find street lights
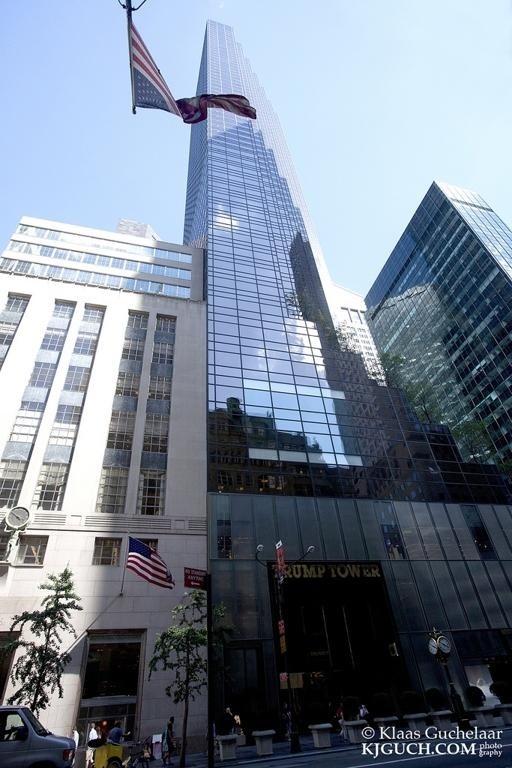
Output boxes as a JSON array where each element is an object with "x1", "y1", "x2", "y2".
[{"x1": 255, "y1": 539, "x2": 318, "y2": 758}]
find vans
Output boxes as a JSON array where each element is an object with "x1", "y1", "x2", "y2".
[{"x1": 0, "y1": 703, "x2": 76, "y2": 767}]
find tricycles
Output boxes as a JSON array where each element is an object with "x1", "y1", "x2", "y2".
[{"x1": 90, "y1": 731, "x2": 152, "y2": 767}]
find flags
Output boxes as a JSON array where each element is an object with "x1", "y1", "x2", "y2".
[{"x1": 125, "y1": 535, "x2": 175, "y2": 592}]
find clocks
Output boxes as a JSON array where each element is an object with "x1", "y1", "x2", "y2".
[
  {"x1": 5, "y1": 506, "x2": 30, "y2": 528},
  {"x1": 427, "y1": 639, "x2": 438, "y2": 656},
  {"x1": 438, "y1": 635, "x2": 452, "y2": 654}
]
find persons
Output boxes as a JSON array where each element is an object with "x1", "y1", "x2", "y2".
[
  {"x1": 72, "y1": 717, "x2": 176, "y2": 768},
  {"x1": 281, "y1": 702, "x2": 294, "y2": 741},
  {"x1": 335, "y1": 702, "x2": 346, "y2": 737},
  {"x1": 359, "y1": 704, "x2": 368, "y2": 720},
  {"x1": 223, "y1": 707, "x2": 243, "y2": 735}
]
